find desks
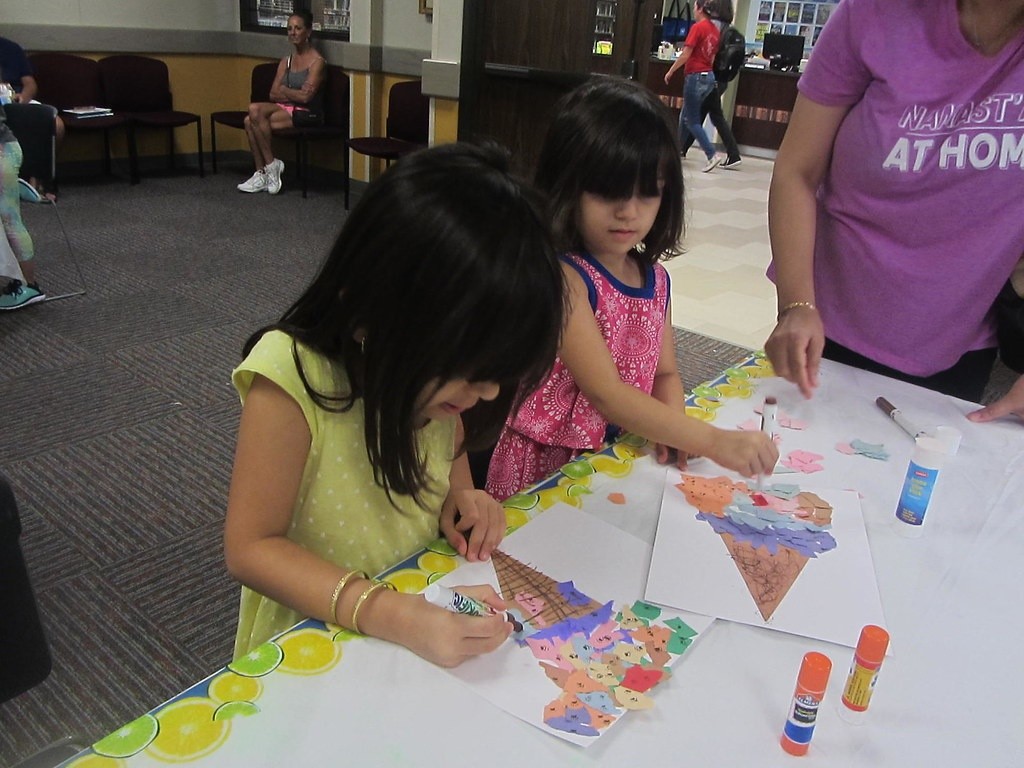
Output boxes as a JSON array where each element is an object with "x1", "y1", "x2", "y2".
[{"x1": 57, "y1": 348, "x2": 1024, "y2": 767}]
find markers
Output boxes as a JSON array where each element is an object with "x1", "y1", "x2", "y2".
[
  {"x1": 425, "y1": 584, "x2": 523, "y2": 633},
  {"x1": 754, "y1": 397, "x2": 778, "y2": 489}
]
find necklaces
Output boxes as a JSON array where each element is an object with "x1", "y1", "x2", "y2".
[{"x1": 971, "y1": 0, "x2": 980, "y2": 47}]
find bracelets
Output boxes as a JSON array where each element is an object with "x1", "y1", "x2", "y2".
[
  {"x1": 352, "y1": 581, "x2": 397, "y2": 637},
  {"x1": 776, "y1": 302, "x2": 816, "y2": 322},
  {"x1": 330, "y1": 568, "x2": 370, "y2": 626}
]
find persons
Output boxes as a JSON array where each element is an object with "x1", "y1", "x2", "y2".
[
  {"x1": 483, "y1": 76, "x2": 779, "y2": 503},
  {"x1": 665, "y1": 0, "x2": 721, "y2": 172},
  {"x1": 224, "y1": 138, "x2": 573, "y2": 667},
  {"x1": 0, "y1": 99, "x2": 46, "y2": 309},
  {"x1": 765, "y1": 0, "x2": 1024, "y2": 425},
  {"x1": 0, "y1": 37, "x2": 64, "y2": 202},
  {"x1": 237, "y1": 10, "x2": 325, "y2": 195},
  {"x1": 680, "y1": 0, "x2": 741, "y2": 168}
]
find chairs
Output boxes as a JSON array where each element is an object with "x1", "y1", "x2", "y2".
[
  {"x1": 2, "y1": 54, "x2": 202, "y2": 305},
  {"x1": 210, "y1": 63, "x2": 430, "y2": 210}
]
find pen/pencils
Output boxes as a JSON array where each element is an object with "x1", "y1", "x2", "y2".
[{"x1": 876, "y1": 395, "x2": 926, "y2": 440}]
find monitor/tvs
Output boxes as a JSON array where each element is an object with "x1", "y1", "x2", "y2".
[{"x1": 763, "y1": 33, "x2": 805, "y2": 70}]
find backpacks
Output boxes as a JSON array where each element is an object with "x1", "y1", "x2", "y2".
[{"x1": 712, "y1": 26, "x2": 745, "y2": 83}]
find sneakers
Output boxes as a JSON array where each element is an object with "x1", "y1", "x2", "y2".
[
  {"x1": 237, "y1": 169, "x2": 269, "y2": 193},
  {"x1": 265, "y1": 158, "x2": 285, "y2": 195},
  {"x1": 17, "y1": 178, "x2": 41, "y2": 203},
  {"x1": 718, "y1": 154, "x2": 743, "y2": 168},
  {"x1": 679, "y1": 150, "x2": 688, "y2": 159},
  {"x1": 0, "y1": 280, "x2": 47, "y2": 310},
  {"x1": 701, "y1": 154, "x2": 721, "y2": 173}
]
